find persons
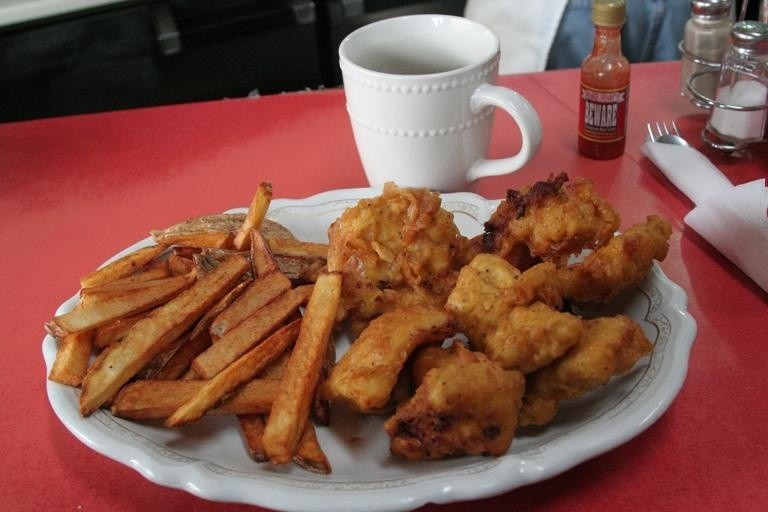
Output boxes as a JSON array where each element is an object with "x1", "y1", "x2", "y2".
[{"x1": 462, "y1": 0, "x2": 692, "y2": 74}]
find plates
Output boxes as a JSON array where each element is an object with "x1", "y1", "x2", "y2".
[{"x1": 43, "y1": 186, "x2": 699, "y2": 512}]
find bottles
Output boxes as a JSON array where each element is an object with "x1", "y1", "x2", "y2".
[
  {"x1": 577, "y1": 1, "x2": 630, "y2": 161},
  {"x1": 682, "y1": 0, "x2": 768, "y2": 144}
]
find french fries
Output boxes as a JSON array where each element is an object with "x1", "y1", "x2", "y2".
[{"x1": 43, "y1": 181, "x2": 344, "y2": 475}]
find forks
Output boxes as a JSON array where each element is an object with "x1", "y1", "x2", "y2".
[{"x1": 646, "y1": 119, "x2": 680, "y2": 143}]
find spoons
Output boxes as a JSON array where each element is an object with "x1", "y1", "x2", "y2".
[{"x1": 659, "y1": 136, "x2": 690, "y2": 149}]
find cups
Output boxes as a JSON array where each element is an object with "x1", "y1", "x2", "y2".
[{"x1": 337, "y1": 14, "x2": 542, "y2": 196}]
